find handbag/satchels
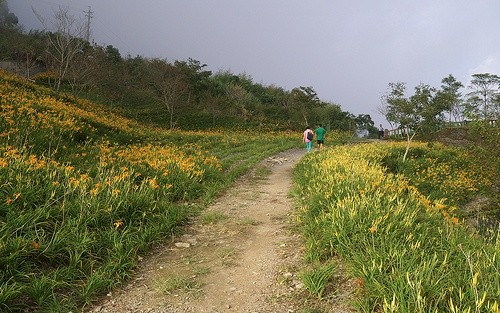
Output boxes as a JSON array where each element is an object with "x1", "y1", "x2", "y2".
[{"x1": 307, "y1": 132, "x2": 314, "y2": 141}]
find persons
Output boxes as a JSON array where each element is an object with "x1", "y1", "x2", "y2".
[
  {"x1": 302, "y1": 126, "x2": 314, "y2": 152},
  {"x1": 377, "y1": 124, "x2": 385, "y2": 139},
  {"x1": 314, "y1": 123, "x2": 326, "y2": 150}
]
